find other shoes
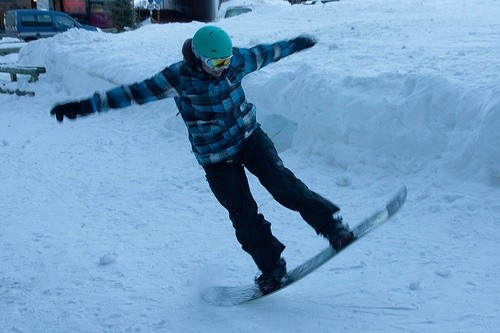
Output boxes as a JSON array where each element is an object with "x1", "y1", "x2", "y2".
[
  {"x1": 320, "y1": 214, "x2": 354, "y2": 253},
  {"x1": 254, "y1": 258, "x2": 288, "y2": 296}
]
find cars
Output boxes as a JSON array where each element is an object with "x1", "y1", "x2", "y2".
[{"x1": 224, "y1": 6, "x2": 253, "y2": 19}]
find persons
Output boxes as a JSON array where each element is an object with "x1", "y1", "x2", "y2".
[{"x1": 51, "y1": 25, "x2": 354, "y2": 295}]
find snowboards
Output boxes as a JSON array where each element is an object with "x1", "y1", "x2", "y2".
[{"x1": 197, "y1": 184, "x2": 409, "y2": 307}]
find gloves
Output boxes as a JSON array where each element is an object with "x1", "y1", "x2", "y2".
[
  {"x1": 50, "y1": 98, "x2": 94, "y2": 121},
  {"x1": 289, "y1": 36, "x2": 316, "y2": 53}
]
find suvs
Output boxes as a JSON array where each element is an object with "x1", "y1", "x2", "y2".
[{"x1": 4, "y1": 9, "x2": 103, "y2": 42}]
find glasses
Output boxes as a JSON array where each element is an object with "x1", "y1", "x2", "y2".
[{"x1": 201, "y1": 55, "x2": 233, "y2": 71}]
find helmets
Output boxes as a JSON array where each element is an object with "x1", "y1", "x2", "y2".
[{"x1": 192, "y1": 25, "x2": 233, "y2": 60}]
